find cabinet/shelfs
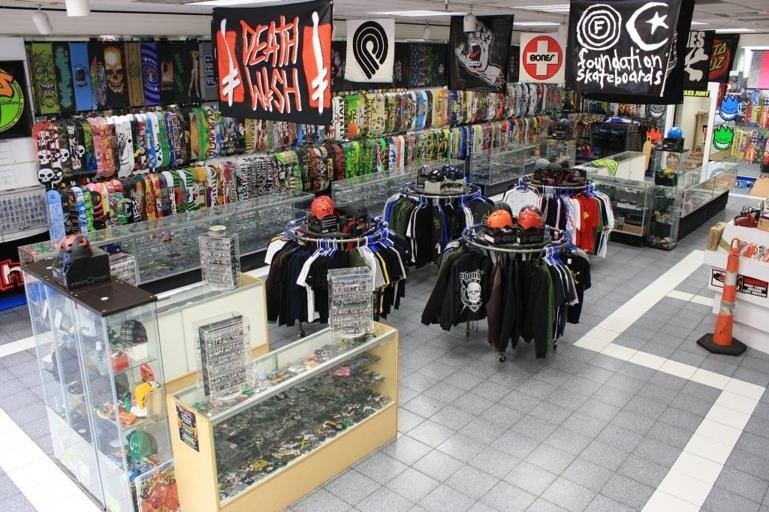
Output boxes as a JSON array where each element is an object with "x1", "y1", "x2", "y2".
[
  {"x1": 702, "y1": 219, "x2": 769, "y2": 356},
  {"x1": 18, "y1": 228, "x2": 139, "y2": 287},
  {"x1": 645, "y1": 187, "x2": 682, "y2": 251},
  {"x1": 573, "y1": 151, "x2": 645, "y2": 181},
  {"x1": 677, "y1": 161, "x2": 739, "y2": 242},
  {"x1": 166, "y1": 321, "x2": 399, "y2": 511},
  {"x1": 654, "y1": 147, "x2": 689, "y2": 187},
  {"x1": 588, "y1": 175, "x2": 654, "y2": 249},
  {"x1": 470, "y1": 142, "x2": 541, "y2": 196},
  {"x1": 330, "y1": 160, "x2": 466, "y2": 216},
  {"x1": 156, "y1": 272, "x2": 270, "y2": 393},
  {"x1": 21, "y1": 261, "x2": 179, "y2": 512},
  {"x1": 116, "y1": 191, "x2": 315, "y2": 285}
]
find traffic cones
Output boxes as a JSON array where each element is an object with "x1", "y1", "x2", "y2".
[{"x1": 692, "y1": 237, "x2": 748, "y2": 356}]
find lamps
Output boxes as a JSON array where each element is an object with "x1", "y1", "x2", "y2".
[
  {"x1": 66, "y1": 0, "x2": 91, "y2": 16},
  {"x1": 463, "y1": 12, "x2": 477, "y2": 34},
  {"x1": 34, "y1": 13, "x2": 52, "y2": 34}
]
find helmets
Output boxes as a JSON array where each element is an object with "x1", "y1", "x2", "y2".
[
  {"x1": 517, "y1": 211, "x2": 544, "y2": 229},
  {"x1": 344, "y1": 202, "x2": 369, "y2": 220},
  {"x1": 557, "y1": 118, "x2": 570, "y2": 129},
  {"x1": 520, "y1": 204, "x2": 542, "y2": 217},
  {"x1": 760, "y1": 154, "x2": 769, "y2": 172},
  {"x1": 428, "y1": 168, "x2": 445, "y2": 182},
  {"x1": 486, "y1": 202, "x2": 513, "y2": 217},
  {"x1": 535, "y1": 158, "x2": 551, "y2": 171},
  {"x1": 547, "y1": 162, "x2": 563, "y2": 173},
  {"x1": 57, "y1": 233, "x2": 92, "y2": 264},
  {"x1": 668, "y1": 126, "x2": 682, "y2": 139},
  {"x1": 446, "y1": 167, "x2": 464, "y2": 179},
  {"x1": 310, "y1": 195, "x2": 335, "y2": 220},
  {"x1": 441, "y1": 164, "x2": 454, "y2": 175},
  {"x1": 486, "y1": 209, "x2": 513, "y2": 228},
  {"x1": 419, "y1": 164, "x2": 433, "y2": 178}
]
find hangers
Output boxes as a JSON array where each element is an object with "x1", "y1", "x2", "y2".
[
  {"x1": 400, "y1": 182, "x2": 489, "y2": 211},
  {"x1": 285, "y1": 216, "x2": 397, "y2": 256},
  {"x1": 457, "y1": 222, "x2": 572, "y2": 265},
  {"x1": 518, "y1": 172, "x2": 596, "y2": 199}
]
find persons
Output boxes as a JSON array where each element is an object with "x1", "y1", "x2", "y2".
[{"x1": 188, "y1": 51, "x2": 200, "y2": 98}]
